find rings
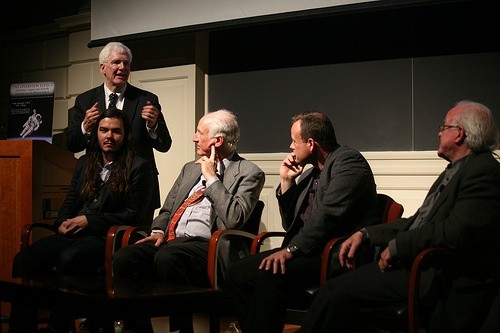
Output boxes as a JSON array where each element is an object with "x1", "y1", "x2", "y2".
[{"x1": 274, "y1": 257, "x2": 279, "y2": 262}]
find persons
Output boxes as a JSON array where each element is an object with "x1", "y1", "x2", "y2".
[
  {"x1": 111, "y1": 109, "x2": 265, "y2": 333},
  {"x1": 225, "y1": 111, "x2": 378, "y2": 333},
  {"x1": 8, "y1": 110, "x2": 156, "y2": 333},
  {"x1": 295, "y1": 100, "x2": 500, "y2": 333},
  {"x1": 64, "y1": 41, "x2": 172, "y2": 230}
]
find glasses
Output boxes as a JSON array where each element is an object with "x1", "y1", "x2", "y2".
[{"x1": 438, "y1": 124, "x2": 458, "y2": 132}]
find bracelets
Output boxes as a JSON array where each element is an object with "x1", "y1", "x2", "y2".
[{"x1": 286, "y1": 244, "x2": 299, "y2": 253}]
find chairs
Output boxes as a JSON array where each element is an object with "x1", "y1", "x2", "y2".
[{"x1": 20, "y1": 193, "x2": 460, "y2": 333}]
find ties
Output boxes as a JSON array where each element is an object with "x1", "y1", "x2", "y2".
[
  {"x1": 107, "y1": 93, "x2": 119, "y2": 109},
  {"x1": 164, "y1": 188, "x2": 207, "y2": 242}
]
